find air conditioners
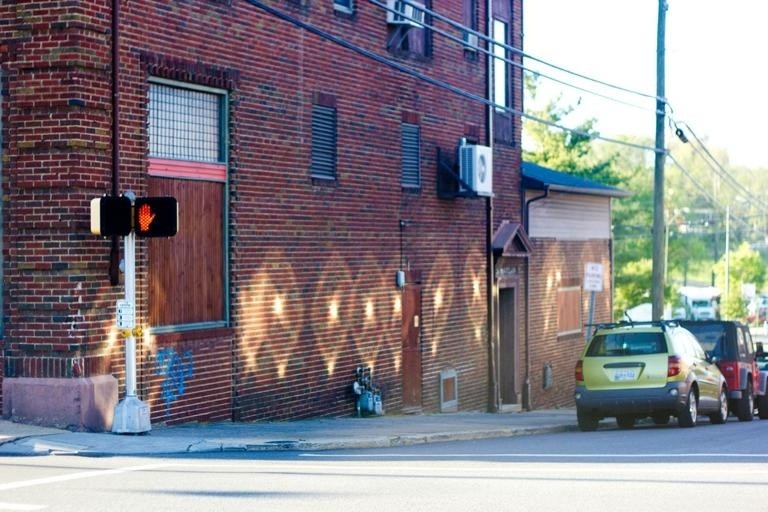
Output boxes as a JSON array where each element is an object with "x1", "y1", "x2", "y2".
[
  {"x1": 459, "y1": 143, "x2": 494, "y2": 193},
  {"x1": 386, "y1": 0, "x2": 426, "y2": 29}
]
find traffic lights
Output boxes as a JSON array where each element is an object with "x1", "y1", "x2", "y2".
[{"x1": 89, "y1": 196, "x2": 179, "y2": 237}]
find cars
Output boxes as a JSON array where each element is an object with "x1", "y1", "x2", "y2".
[{"x1": 575, "y1": 319, "x2": 768, "y2": 427}]
